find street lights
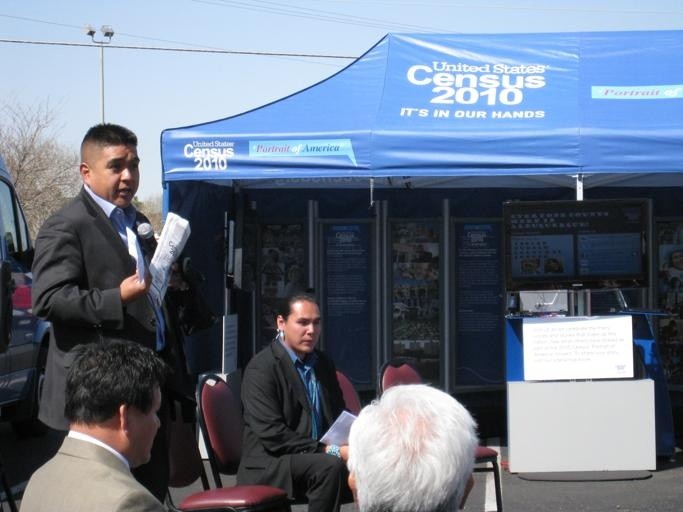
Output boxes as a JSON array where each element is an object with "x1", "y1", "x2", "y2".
[{"x1": 86, "y1": 23, "x2": 113, "y2": 125}]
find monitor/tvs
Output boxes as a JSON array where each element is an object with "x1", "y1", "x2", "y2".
[
  {"x1": 228, "y1": 190, "x2": 259, "y2": 292},
  {"x1": 501, "y1": 197, "x2": 654, "y2": 291}
]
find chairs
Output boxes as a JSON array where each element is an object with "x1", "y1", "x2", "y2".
[{"x1": 158, "y1": 362, "x2": 501, "y2": 512}]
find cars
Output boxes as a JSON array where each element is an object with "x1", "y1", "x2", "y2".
[{"x1": 0, "y1": 156, "x2": 51, "y2": 435}]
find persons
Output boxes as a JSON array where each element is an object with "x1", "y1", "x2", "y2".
[
  {"x1": 32, "y1": 124, "x2": 182, "y2": 505},
  {"x1": 18, "y1": 342, "x2": 170, "y2": 511},
  {"x1": 657, "y1": 244, "x2": 683, "y2": 386},
  {"x1": 346, "y1": 383, "x2": 481, "y2": 511},
  {"x1": 236, "y1": 292, "x2": 349, "y2": 512}
]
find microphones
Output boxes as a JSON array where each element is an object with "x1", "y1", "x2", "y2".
[{"x1": 137, "y1": 222, "x2": 157, "y2": 253}]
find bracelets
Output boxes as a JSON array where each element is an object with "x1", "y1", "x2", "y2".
[{"x1": 326, "y1": 444, "x2": 341, "y2": 457}]
